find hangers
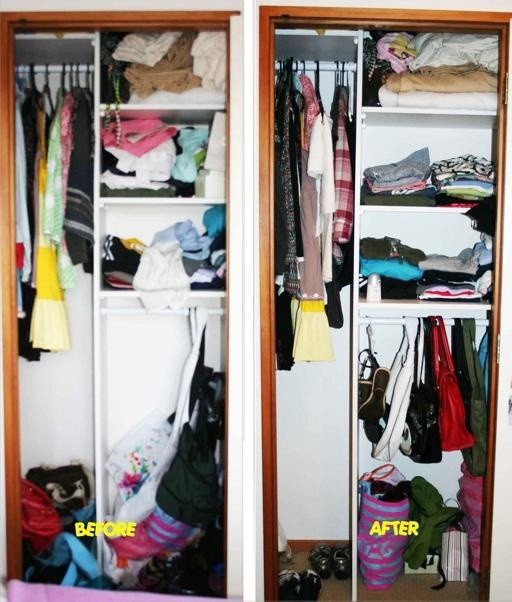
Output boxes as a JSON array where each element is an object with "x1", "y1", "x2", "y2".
[
  {"x1": 13, "y1": 62, "x2": 94, "y2": 129},
  {"x1": 273, "y1": 57, "x2": 356, "y2": 138}
]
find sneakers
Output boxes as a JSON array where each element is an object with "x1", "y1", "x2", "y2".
[
  {"x1": 309, "y1": 544, "x2": 332, "y2": 577},
  {"x1": 335, "y1": 543, "x2": 351, "y2": 579},
  {"x1": 278, "y1": 569, "x2": 299, "y2": 600},
  {"x1": 301, "y1": 570, "x2": 321, "y2": 600}
]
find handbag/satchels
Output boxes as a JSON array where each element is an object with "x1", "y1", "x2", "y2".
[
  {"x1": 442, "y1": 521, "x2": 469, "y2": 583},
  {"x1": 145, "y1": 505, "x2": 193, "y2": 547},
  {"x1": 402, "y1": 478, "x2": 465, "y2": 590},
  {"x1": 462, "y1": 317, "x2": 487, "y2": 476},
  {"x1": 354, "y1": 347, "x2": 390, "y2": 422},
  {"x1": 155, "y1": 321, "x2": 221, "y2": 521},
  {"x1": 454, "y1": 462, "x2": 482, "y2": 575},
  {"x1": 105, "y1": 410, "x2": 177, "y2": 500},
  {"x1": 430, "y1": 316, "x2": 475, "y2": 452},
  {"x1": 357, "y1": 494, "x2": 409, "y2": 590},
  {"x1": 410, "y1": 317, "x2": 442, "y2": 464},
  {"x1": 374, "y1": 316, "x2": 419, "y2": 463},
  {"x1": 23, "y1": 460, "x2": 90, "y2": 512}
]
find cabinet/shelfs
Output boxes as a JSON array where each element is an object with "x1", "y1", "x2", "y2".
[
  {"x1": 265, "y1": 8, "x2": 509, "y2": 601},
  {"x1": 8, "y1": 12, "x2": 240, "y2": 601}
]
